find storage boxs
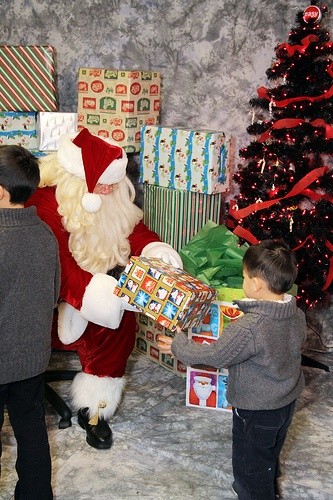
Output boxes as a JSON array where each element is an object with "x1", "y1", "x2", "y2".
[
  {"x1": 142, "y1": 184, "x2": 222, "y2": 254},
  {"x1": 0, "y1": 44, "x2": 60, "y2": 112},
  {"x1": 77, "y1": 67, "x2": 162, "y2": 153},
  {"x1": 137, "y1": 125, "x2": 231, "y2": 196},
  {"x1": 1, "y1": 108, "x2": 49, "y2": 158},
  {"x1": 112, "y1": 256, "x2": 220, "y2": 334},
  {"x1": 37, "y1": 111, "x2": 78, "y2": 151}
]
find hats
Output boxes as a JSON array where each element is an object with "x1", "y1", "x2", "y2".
[{"x1": 57, "y1": 128, "x2": 128, "y2": 213}]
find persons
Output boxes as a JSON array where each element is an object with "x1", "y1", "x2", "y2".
[
  {"x1": 156, "y1": 238, "x2": 307, "y2": 500},
  {"x1": 23, "y1": 128, "x2": 180, "y2": 452},
  {"x1": 0, "y1": 145, "x2": 59, "y2": 499}
]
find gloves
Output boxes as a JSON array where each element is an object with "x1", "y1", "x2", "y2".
[
  {"x1": 122, "y1": 299, "x2": 139, "y2": 314},
  {"x1": 142, "y1": 242, "x2": 183, "y2": 270}
]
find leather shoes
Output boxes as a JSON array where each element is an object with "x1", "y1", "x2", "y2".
[{"x1": 77, "y1": 404, "x2": 113, "y2": 448}]
